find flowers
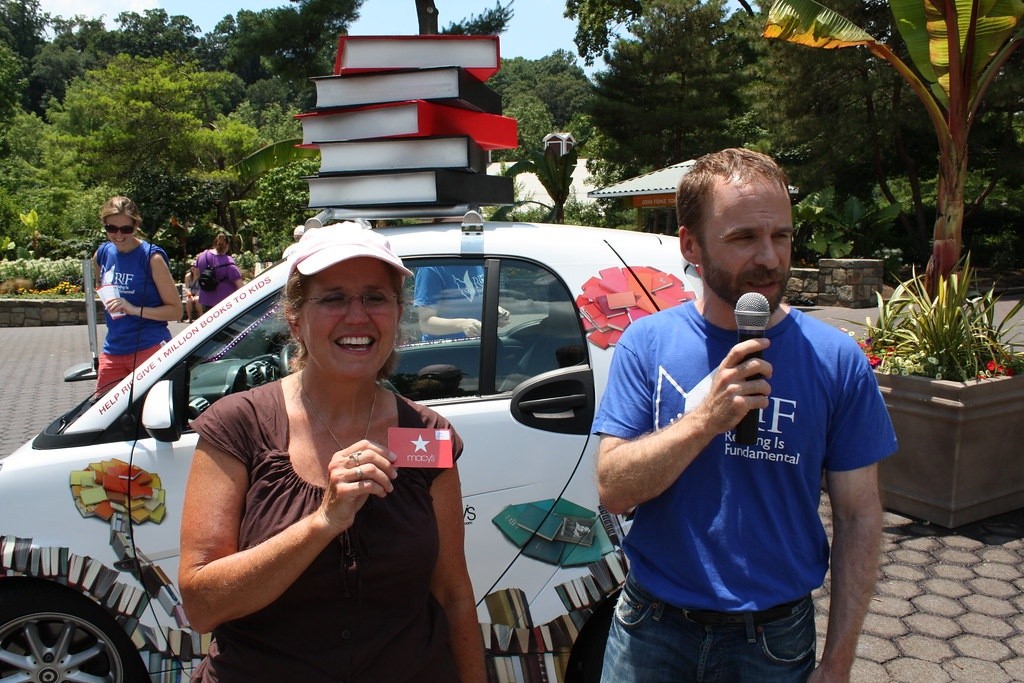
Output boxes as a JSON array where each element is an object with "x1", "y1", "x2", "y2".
[{"x1": 761, "y1": 1, "x2": 1023, "y2": 387}]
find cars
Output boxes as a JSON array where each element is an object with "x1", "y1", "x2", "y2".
[{"x1": 1, "y1": 208, "x2": 710, "y2": 683}]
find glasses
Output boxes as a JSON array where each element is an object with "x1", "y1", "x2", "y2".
[{"x1": 104, "y1": 223, "x2": 137, "y2": 234}]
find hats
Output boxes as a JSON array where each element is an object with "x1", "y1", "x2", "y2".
[
  {"x1": 285, "y1": 219, "x2": 415, "y2": 296},
  {"x1": 293, "y1": 224, "x2": 304, "y2": 235}
]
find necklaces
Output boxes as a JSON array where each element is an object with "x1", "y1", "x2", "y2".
[{"x1": 301, "y1": 371, "x2": 377, "y2": 449}]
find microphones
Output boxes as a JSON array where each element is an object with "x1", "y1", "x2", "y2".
[{"x1": 734, "y1": 292, "x2": 771, "y2": 446}]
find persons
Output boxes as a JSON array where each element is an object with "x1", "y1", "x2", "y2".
[
  {"x1": 413, "y1": 218, "x2": 510, "y2": 340},
  {"x1": 184, "y1": 261, "x2": 203, "y2": 323},
  {"x1": 94, "y1": 196, "x2": 183, "y2": 397},
  {"x1": 283, "y1": 225, "x2": 305, "y2": 258},
  {"x1": 179, "y1": 222, "x2": 488, "y2": 683},
  {"x1": 591, "y1": 149, "x2": 900, "y2": 683},
  {"x1": 419, "y1": 364, "x2": 467, "y2": 396},
  {"x1": 195, "y1": 233, "x2": 243, "y2": 313}
]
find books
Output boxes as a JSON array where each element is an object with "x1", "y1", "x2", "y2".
[{"x1": 294, "y1": 34, "x2": 518, "y2": 209}]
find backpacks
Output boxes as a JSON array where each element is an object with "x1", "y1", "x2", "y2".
[{"x1": 198, "y1": 250, "x2": 236, "y2": 291}]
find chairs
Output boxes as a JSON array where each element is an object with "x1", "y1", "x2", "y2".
[{"x1": 499, "y1": 305, "x2": 586, "y2": 394}]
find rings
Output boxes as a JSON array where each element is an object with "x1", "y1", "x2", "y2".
[
  {"x1": 349, "y1": 451, "x2": 361, "y2": 466},
  {"x1": 356, "y1": 466, "x2": 362, "y2": 479}
]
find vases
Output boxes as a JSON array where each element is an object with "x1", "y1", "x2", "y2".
[{"x1": 867, "y1": 369, "x2": 1024, "y2": 530}]
